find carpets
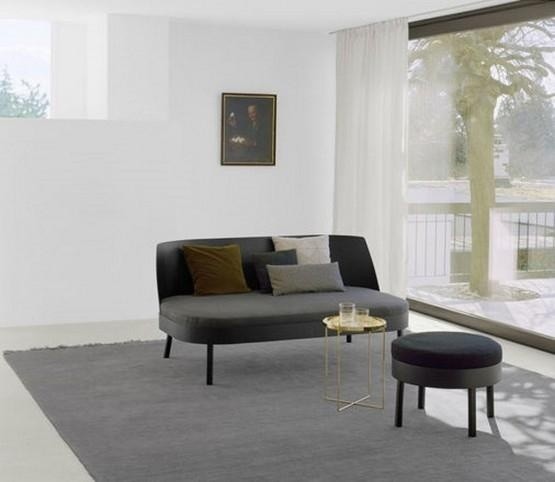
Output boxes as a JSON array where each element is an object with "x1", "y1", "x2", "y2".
[{"x1": 2, "y1": 327, "x2": 555, "y2": 482}]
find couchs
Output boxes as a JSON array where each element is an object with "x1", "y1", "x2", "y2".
[{"x1": 156, "y1": 234, "x2": 410, "y2": 386}]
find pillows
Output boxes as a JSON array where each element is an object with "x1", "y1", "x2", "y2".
[{"x1": 182, "y1": 235, "x2": 347, "y2": 297}]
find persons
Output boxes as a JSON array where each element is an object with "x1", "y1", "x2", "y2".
[{"x1": 227, "y1": 104, "x2": 267, "y2": 163}]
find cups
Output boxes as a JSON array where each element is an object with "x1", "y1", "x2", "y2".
[{"x1": 339, "y1": 303, "x2": 370, "y2": 325}]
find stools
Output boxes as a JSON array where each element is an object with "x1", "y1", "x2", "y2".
[
  {"x1": 322, "y1": 315, "x2": 388, "y2": 413},
  {"x1": 391, "y1": 331, "x2": 502, "y2": 437}
]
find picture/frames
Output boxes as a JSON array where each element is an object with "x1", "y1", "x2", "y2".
[{"x1": 221, "y1": 93, "x2": 277, "y2": 166}]
